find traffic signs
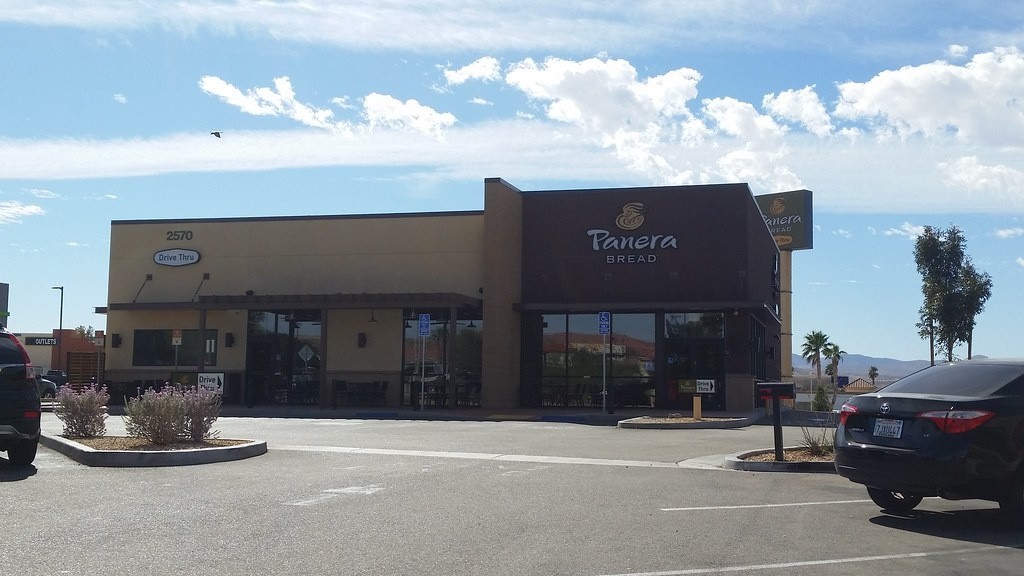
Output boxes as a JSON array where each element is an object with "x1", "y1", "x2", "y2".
[{"x1": 696, "y1": 380, "x2": 715, "y2": 393}]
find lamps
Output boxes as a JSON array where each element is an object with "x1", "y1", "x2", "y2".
[
  {"x1": 368, "y1": 302, "x2": 378, "y2": 323},
  {"x1": 112, "y1": 333, "x2": 121, "y2": 348},
  {"x1": 404, "y1": 320, "x2": 412, "y2": 329},
  {"x1": 132, "y1": 273, "x2": 153, "y2": 306},
  {"x1": 224, "y1": 333, "x2": 235, "y2": 347},
  {"x1": 190, "y1": 273, "x2": 210, "y2": 307},
  {"x1": 358, "y1": 333, "x2": 365, "y2": 346},
  {"x1": 467, "y1": 319, "x2": 477, "y2": 328}
]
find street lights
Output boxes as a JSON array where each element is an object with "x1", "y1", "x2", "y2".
[
  {"x1": 52, "y1": 285, "x2": 64, "y2": 389},
  {"x1": 808, "y1": 371, "x2": 815, "y2": 412}
]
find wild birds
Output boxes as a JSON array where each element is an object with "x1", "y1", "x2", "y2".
[{"x1": 211, "y1": 132, "x2": 222, "y2": 138}]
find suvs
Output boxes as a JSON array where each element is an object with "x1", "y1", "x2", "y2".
[{"x1": 0, "y1": 325, "x2": 43, "y2": 467}]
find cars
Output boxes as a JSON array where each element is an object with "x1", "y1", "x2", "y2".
[
  {"x1": 36, "y1": 378, "x2": 58, "y2": 398},
  {"x1": 602, "y1": 374, "x2": 653, "y2": 408},
  {"x1": 830, "y1": 357, "x2": 1024, "y2": 513},
  {"x1": 403, "y1": 358, "x2": 480, "y2": 397}
]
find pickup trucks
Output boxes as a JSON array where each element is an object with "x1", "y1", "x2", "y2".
[{"x1": 42, "y1": 370, "x2": 67, "y2": 380}]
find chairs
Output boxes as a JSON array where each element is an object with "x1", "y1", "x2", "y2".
[
  {"x1": 292, "y1": 378, "x2": 319, "y2": 406},
  {"x1": 541, "y1": 383, "x2": 638, "y2": 406},
  {"x1": 332, "y1": 380, "x2": 388, "y2": 404},
  {"x1": 410, "y1": 379, "x2": 482, "y2": 412}
]
